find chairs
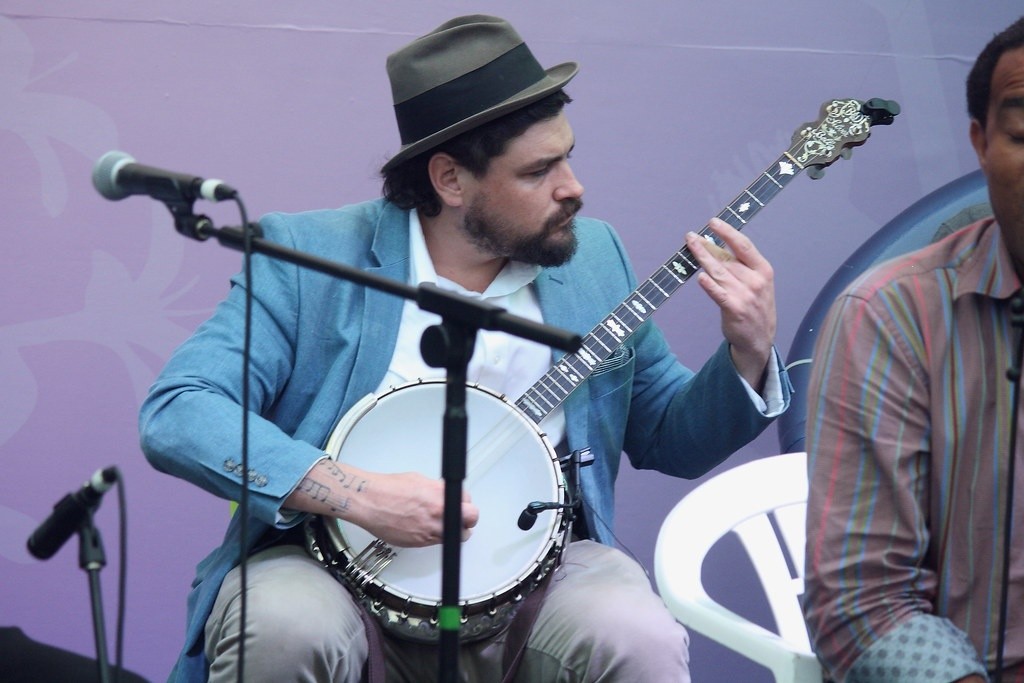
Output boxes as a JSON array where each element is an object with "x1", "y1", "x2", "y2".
[{"x1": 655, "y1": 453, "x2": 822, "y2": 683}]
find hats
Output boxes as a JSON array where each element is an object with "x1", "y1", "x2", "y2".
[{"x1": 381, "y1": 14, "x2": 579, "y2": 173}]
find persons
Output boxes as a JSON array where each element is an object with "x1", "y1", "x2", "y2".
[
  {"x1": 136, "y1": 14, "x2": 796, "y2": 682},
  {"x1": 797, "y1": 13, "x2": 1024, "y2": 683}
]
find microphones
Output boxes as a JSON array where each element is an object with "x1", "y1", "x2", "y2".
[
  {"x1": 26, "y1": 466, "x2": 118, "y2": 560},
  {"x1": 518, "y1": 501, "x2": 547, "y2": 530},
  {"x1": 92, "y1": 150, "x2": 237, "y2": 201}
]
